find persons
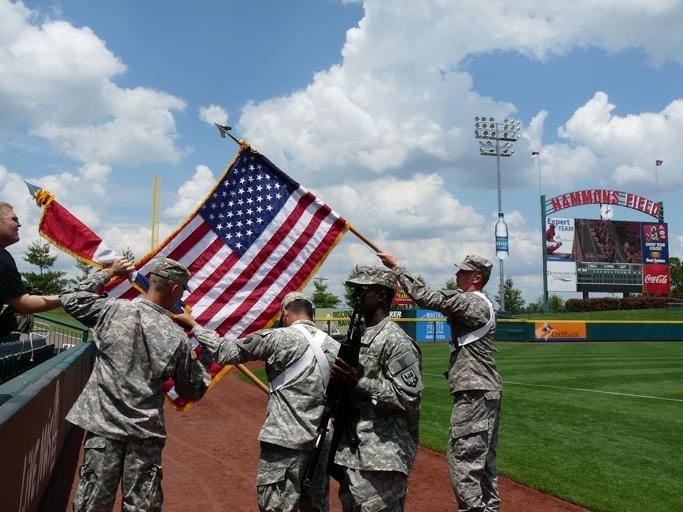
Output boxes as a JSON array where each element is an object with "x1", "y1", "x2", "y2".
[
  {"x1": 377, "y1": 250, "x2": 503, "y2": 511},
  {"x1": 330, "y1": 263, "x2": 426, "y2": 512},
  {"x1": 0, "y1": 203, "x2": 63, "y2": 344},
  {"x1": 546, "y1": 224, "x2": 562, "y2": 254},
  {"x1": 61, "y1": 256, "x2": 214, "y2": 512},
  {"x1": 172, "y1": 290, "x2": 340, "y2": 512}
]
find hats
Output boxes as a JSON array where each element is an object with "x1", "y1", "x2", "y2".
[
  {"x1": 454, "y1": 254, "x2": 493, "y2": 272},
  {"x1": 149, "y1": 257, "x2": 191, "y2": 293},
  {"x1": 279, "y1": 291, "x2": 312, "y2": 327},
  {"x1": 345, "y1": 265, "x2": 401, "y2": 291}
]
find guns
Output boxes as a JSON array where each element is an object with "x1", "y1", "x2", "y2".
[{"x1": 301, "y1": 286, "x2": 368, "y2": 488}]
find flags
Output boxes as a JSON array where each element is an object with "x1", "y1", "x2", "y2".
[
  {"x1": 91, "y1": 143, "x2": 347, "y2": 413},
  {"x1": 34, "y1": 189, "x2": 194, "y2": 338}
]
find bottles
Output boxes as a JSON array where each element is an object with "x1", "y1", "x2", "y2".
[{"x1": 494, "y1": 212, "x2": 512, "y2": 260}]
